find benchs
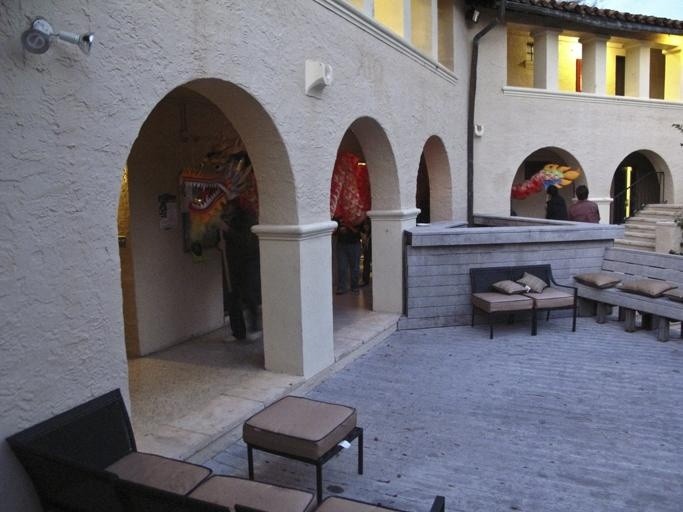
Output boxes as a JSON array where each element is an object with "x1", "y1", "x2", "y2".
[
  {"x1": 0, "y1": 388, "x2": 448, "y2": 511},
  {"x1": 573, "y1": 248, "x2": 682, "y2": 342},
  {"x1": 471, "y1": 264, "x2": 578, "y2": 339}
]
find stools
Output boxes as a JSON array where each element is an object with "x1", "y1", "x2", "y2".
[{"x1": 241, "y1": 393, "x2": 364, "y2": 501}]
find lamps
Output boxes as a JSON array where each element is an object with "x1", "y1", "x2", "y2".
[{"x1": 21, "y1": 16, "x2": 91, "y2": 56}]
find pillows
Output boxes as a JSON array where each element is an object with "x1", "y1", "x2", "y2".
[
  {"x1": 573, "y1": 272, "x2": 620, "y2": 288},
  {"x1": 616, "y1": 278, "x2": 678, "y2": 297},
  {"x1": 492, "y1": 280, "x2": 527, "y2": 294},
  {"x1": 516, "y1": 271, "x2": 547, "y2": 294},
  {"x1": 663, "y1": 287, "x2": 682, "y2": 301}
]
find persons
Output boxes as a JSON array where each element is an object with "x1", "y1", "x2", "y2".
[
  {"x1": 335, "y1": 219, "x2": 360, "y2": 294},
  {"x1": 215, "y1": 200, "x2": 263, "y2": 344},
  {"x1": 546, "y1": 185, "x2": 566, "y2": 220},
  {"x1": 358, "y1": 219, "x2": 373, "y2": 288},
  {"x1": 570, "y1": 186, "x2": 600, "y2": 224}
]
